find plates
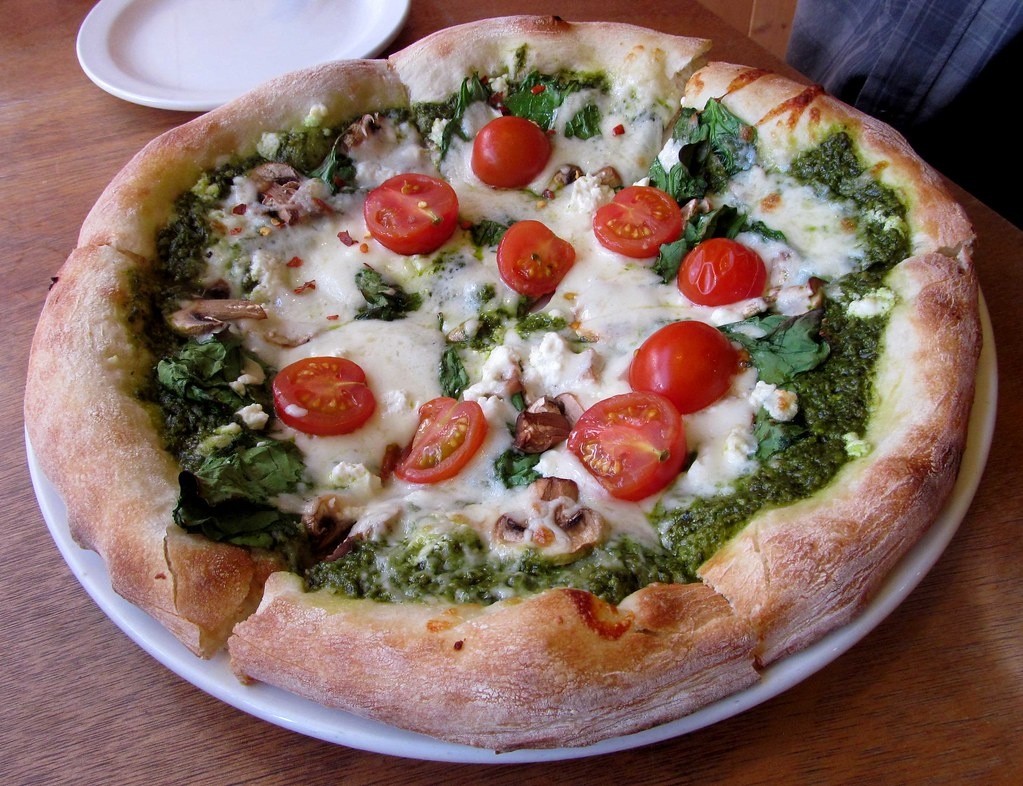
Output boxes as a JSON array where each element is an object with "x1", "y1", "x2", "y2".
[
  {"x1": 76, "y1": 0, "x2": 413, "y2": 111},
  {"x1": 24, "y1": 39, "x2": 1002, "y2": 763}
]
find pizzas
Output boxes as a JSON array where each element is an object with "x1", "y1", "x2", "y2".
[{"x1": 22, "y1": 14, "x2": 980, "y2": 752}]
